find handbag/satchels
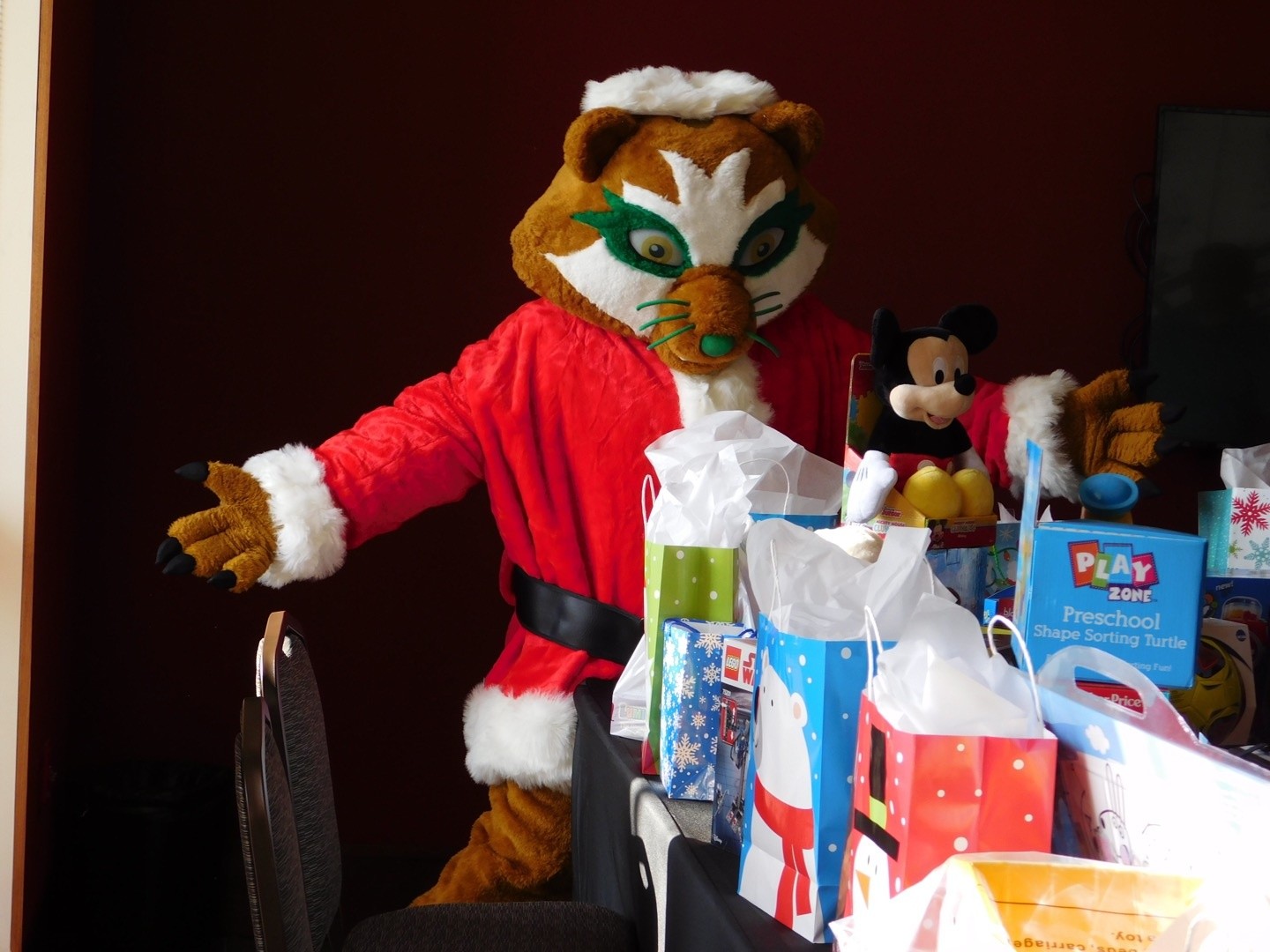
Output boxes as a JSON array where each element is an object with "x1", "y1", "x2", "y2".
[{"x1": 610, "y1": 409, "x2": 1270, "y2": 952}]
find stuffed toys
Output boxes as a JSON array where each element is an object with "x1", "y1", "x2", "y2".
[
  {"x1": 829, "y1": 297, "x2": 1011, "y2": 525},
  {"x1": 178, "y1": 48, "x2": 1168, "y2": 916}
]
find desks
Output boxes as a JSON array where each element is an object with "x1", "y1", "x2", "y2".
[{"x1": 571, "y1": 683, "x2": 833, "y2": 952}]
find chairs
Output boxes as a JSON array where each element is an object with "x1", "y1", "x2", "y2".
[
  {"x1": 255, "y1": 608, "x2": 627, "y2": 951},
  {"x1": 233, "y1": 695, "x2": 320, "y2": 951}
]
